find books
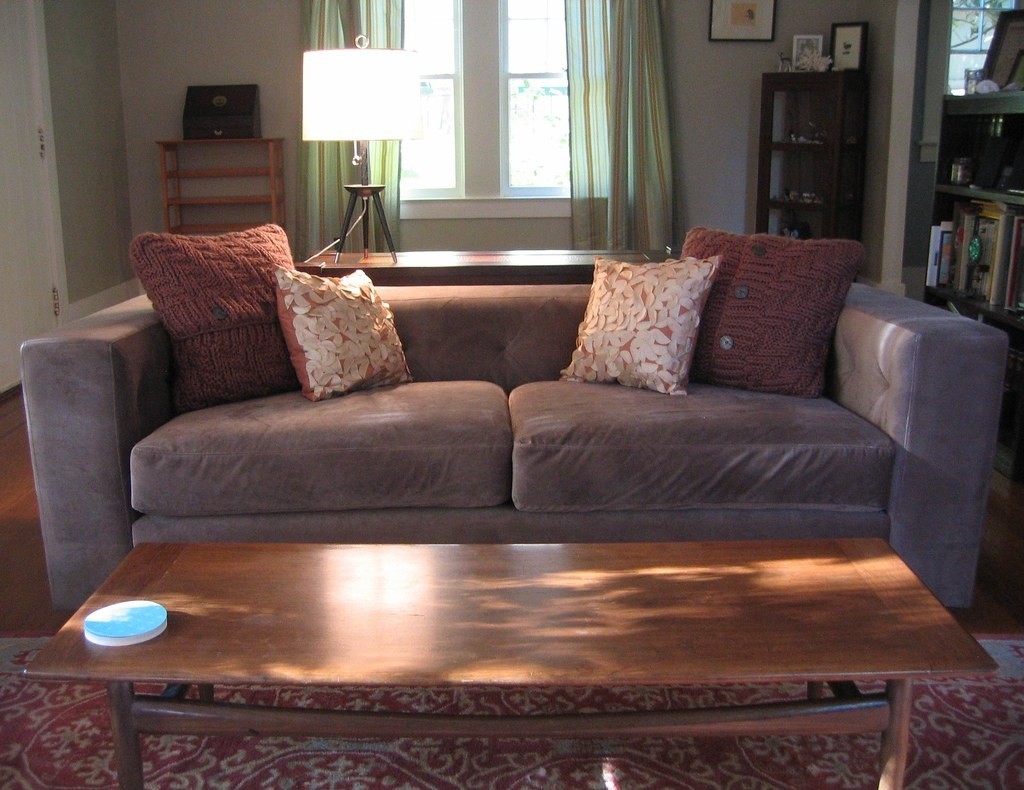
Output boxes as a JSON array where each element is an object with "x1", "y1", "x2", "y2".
[{"x1": 925, "y1": 198, "x2": 1024, "y2": 314}]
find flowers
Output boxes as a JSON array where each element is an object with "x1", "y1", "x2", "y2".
[{"x1": 797, "y1": 48, "x2": 833, "y2": 71}]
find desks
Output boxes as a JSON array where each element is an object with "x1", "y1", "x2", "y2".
[{"x1": 301, "y1": 245, "x2": 683, "y2": 285}]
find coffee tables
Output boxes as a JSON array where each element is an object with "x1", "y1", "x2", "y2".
[{"x1": 17, "y1": 537, "x2": 1005, "y2": 790}]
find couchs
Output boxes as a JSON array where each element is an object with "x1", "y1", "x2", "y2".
[{"x1": 18, "y1": 279, "x2": 1009, "y2": 626}]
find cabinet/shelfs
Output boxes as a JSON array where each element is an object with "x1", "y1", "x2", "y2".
[
  {"x1": 752, "y1": 70, "x2": 870, "y2": 240},
  {"x1": 156, "y1": 136, "x2": 287, "y2": 234},
  {"x1": 923, "y1": 90, "x2": 1024, "y2": 482}
]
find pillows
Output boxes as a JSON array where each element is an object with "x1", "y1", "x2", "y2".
[
  {"x1": 133, "y1": 224, "x2": 412, "y2": 411},
  {"x1": 565, "y1": 226, "x2": 869, "y2": 398}
]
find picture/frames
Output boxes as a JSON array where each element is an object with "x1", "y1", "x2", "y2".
[
  {"x1": 707, "y1": 0, "x2": 776, "y2": 42},
  {"x1": 976, "y1": 9, "x2": 1024, "y2": 91},
  {"x1": 791, "y1": 34, "x2": 824, "y2": 71},
  {"x1": 831, "y1": 21, "x2": 867, "y2": 70}
]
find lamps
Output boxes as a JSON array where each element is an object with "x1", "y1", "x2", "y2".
[{"x1": 297, "y1": 37, "x2": 422, "y2": 263}]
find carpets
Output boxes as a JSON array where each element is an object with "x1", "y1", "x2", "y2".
[{"x1": 0, "y1": 615, "x2": 1024, "y2": 790}]
